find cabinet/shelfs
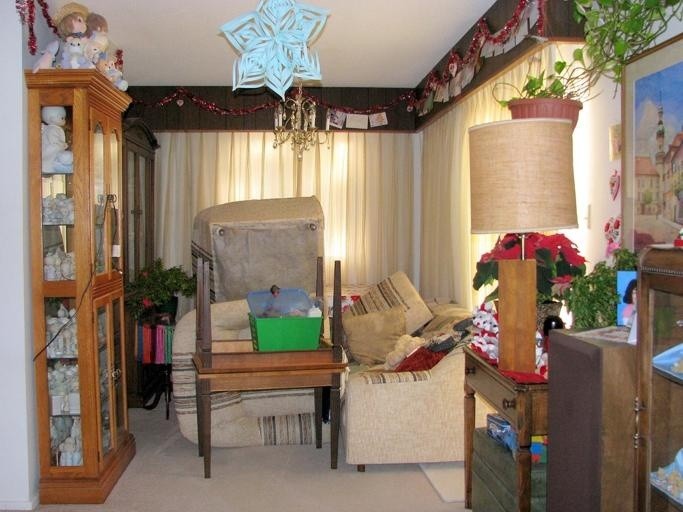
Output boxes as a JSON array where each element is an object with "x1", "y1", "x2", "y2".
[
  {"x1": 633, "y1": 243, "x2": 683, "y2": 512},
  {"x1": 25, "y1": 68, "x2": 137, "y2": 505},
  {"x1": 546, "y1": 325, "x2": 639, "y2": 511},
  {"x1": 123, "y1": 119, "x2": 161, "y2": 408}
]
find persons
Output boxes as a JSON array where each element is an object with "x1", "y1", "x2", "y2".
[{"x1": 623, "y1": 278, "x2": 637, "y2": 323}]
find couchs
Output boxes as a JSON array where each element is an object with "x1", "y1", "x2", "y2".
[{"x1": 172, "y1": 284, "x2": 489, "y2": 471}]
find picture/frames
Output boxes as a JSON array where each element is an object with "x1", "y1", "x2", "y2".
[{"x1": 620, "y1": 32, "x2": 683, "y2": 256}]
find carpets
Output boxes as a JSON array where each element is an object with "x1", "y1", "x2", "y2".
[{"x1": 418, "y1": 463, "x2": 466, "y2": 504}]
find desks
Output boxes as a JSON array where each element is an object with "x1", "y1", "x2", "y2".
[
  {"x1": 189, "y1": 256, "x2": 347, "y2": 478},
  {"x1": 462, "y1": 347, "x2": 549, "y2": 512}
]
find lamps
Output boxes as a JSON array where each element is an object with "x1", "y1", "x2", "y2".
[
  {"x1": 272, "y1": 79, "x2": 332, "y2": 160},
  {"x1": 469, "y1": 117, "x2": 578, "y2": 374}
]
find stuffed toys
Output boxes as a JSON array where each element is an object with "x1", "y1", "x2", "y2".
[
  {"x1": 470, "y1": 302, "x2": 566, "y2": 384},
  {"x1": 31, "y1": 106, "x2": 113, "y2": 466},
  {"x1": 263, "y1": 284, "x2": 280, "y2": 317},
  {"x1": 382, "y1": 333, "x2": 425, "y2": 371},
  {"x1": 31, "y1": 2, "x2": 130, "y2": 94}
]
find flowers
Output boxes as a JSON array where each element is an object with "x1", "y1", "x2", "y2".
[
  {"x1": 473, "y1": 231, "x2": 589, "y2": 304},
  {"x1": 126, "y1": 256, "x2": 195, "y2": 318}
]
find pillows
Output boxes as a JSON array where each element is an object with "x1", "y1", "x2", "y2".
[
  {"x1": 342, "y1": 269, "x2": 434, "y2": 335},
  {"x1": 342, "y1": 307, "x2": 407, "y2": 366}
]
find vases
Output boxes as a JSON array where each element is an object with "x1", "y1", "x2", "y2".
[
  {"x1": 152, "y1": 298, "x2": 177, "y2": 325},
  {"x1": 495, "y1": 300, "x2": 563, "y2": 331}
]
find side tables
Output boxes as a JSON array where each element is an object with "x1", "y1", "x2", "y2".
[{"x1": 143, "y1": 296, "x2": 176, "y2": 420}]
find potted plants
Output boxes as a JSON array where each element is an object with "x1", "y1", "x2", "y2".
[{"x1": 492, "y1": 0, "x2": 683, "y2": 131}]
